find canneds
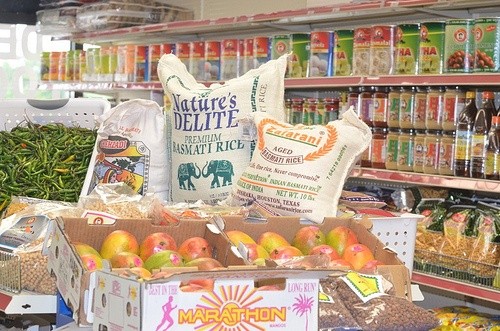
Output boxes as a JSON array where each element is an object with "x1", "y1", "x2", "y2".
[{"x1": 285, "y1": 85, "x2": 467, "y2": 178}]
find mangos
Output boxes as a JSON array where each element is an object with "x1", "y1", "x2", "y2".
[
  {"x1": 223, "y1": 225, "x2": 387, "y2": 272},
  {"x1": 73, "y1": 230, "x2": 225, "y2": 280}
]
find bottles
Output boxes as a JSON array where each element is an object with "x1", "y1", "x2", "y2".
[
  {"x1": 492, "y1": 104, "x2": 500, "y2": 180},
  {"x1": 455, "y1": 91, "x2": 478, "y2": 177},
  {"x1": 469, "y1": 90, "x2": 497, "y2": 179},
  {"x1": 286, "y1": 87, "x2": 466, "y2": 175}
]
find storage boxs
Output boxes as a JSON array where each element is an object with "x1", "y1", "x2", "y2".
[
  {"x1": 0, "y1": 98, "x2": 112, "y2": 207},
  {"x1": 42, "y1": 212, "x2": 425, "y2": 331}
]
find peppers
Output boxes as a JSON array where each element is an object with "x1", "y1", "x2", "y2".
[{"x1": 0, "y1": 114, "x2": 97, "y2": 217}]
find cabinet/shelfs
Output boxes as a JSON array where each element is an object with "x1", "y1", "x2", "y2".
[{"x1": 37, "y1": 0, "x2": 500, "y2": 315}]
